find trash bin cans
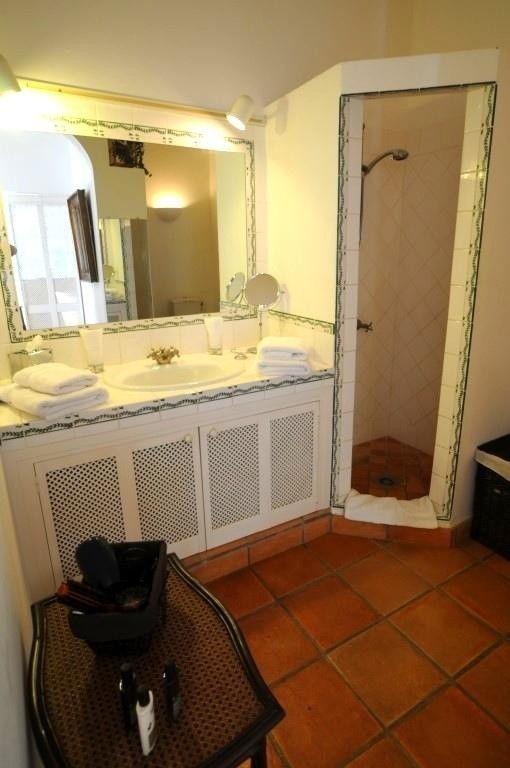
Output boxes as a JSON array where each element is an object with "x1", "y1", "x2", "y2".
[{"x1": 470, "y1": 432, "x2": 510, "y2": 561}]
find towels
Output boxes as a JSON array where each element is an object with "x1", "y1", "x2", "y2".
[
  {"x1": 2, "y1": 384, "x2": 110, "y2": 420},
  {"x1": 13, "y1": 361, "x2": 96, "y2": 396},
  {"x1": 257, "y1": 333, "x2": 306, "y2": 357},
  {"x1": 105, "y1": 287, "x2": 118, "y2": 296},
  {"x1": 345, "y1": 487, "x2": 438, "y2": 530},
  {"x1": 257, "y1": 356, "x2": 311, "y2": 379}
]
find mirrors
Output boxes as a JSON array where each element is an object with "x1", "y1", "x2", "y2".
[
  {"x1": 0, "y1": 109, "x2": 256, "y2": 343},
  {"x1": 96, "y1": 217, "x2": 154, "y2": 324},
  {"x1": 103, "y1": 263, "x2": 115, "y2": 284},
  {"x1": 241, "y1": 271, "x2": 282, "y2": 351},
  {"x1": 217, "y1": 270, "x2": 244, "y2": 311}
]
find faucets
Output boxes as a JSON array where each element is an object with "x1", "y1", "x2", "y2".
[{"x1": 147, "y1": 346, "x2": 182, "y2": 365}]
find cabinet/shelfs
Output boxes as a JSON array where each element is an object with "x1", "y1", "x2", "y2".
[{"x1": 33, "y1": 376, "x2": 336, "y2": 586}]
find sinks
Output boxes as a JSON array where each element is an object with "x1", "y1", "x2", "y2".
[{"x1": 103, "y1": 356, "x2": 244, "y2": 391}]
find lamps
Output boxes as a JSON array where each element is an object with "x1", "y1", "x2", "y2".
[
  {"x1": 0, "y1": 51, "x2": 20, "y2": 100},
  {"x1": 226, "y1": 94, "x2": 257, "y2": 131},
  {"x1": 150, "y1": 193, "x2": 184, "y2": 222}
]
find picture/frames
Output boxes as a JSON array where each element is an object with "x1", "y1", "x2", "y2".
[
  {"x1": 109, "y1": 139, "x2": 140, "y2": 169},
  {"x1": 66, "y1": 189, "x2": 100, "y2": 283}
]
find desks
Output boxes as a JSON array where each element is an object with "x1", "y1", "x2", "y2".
[{"x1": 30, "y1": 556, "x2": 285, "y2": 766}]
